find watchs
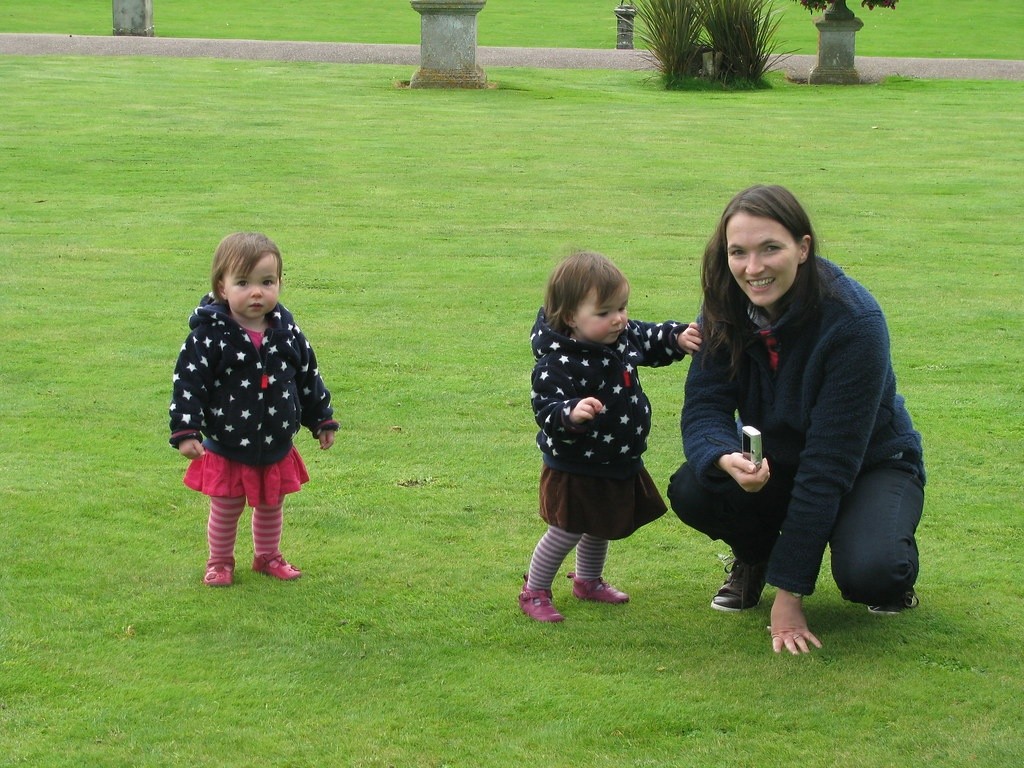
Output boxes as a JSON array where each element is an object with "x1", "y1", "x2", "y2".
[{"x1": 791, "y1": 593, "x2": 802, "y2": 598}]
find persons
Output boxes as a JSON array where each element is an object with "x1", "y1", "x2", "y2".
[
  {"x1": 517, "y1": 251, "x2": 704, "y2": 623},
  {"x1": 666, "y1": 182, "x2": 931, "y2": 660},
  {"x1": 167, "y1": 230, "x2": 340, "y2": 587}
]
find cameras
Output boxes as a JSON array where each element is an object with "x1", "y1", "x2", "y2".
[{"x1": 742, "y1": 426, "x2": 763, "y2": 472}]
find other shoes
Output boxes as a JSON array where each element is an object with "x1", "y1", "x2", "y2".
[
  {"x1": 518, "y1": 574, "x2": 563, "y2": 622},
  {"x1": 567, "y1": 570, "x2": 629, "y2": 603},
  {"x1": 252, "y1": 551, "x2": 301, "y2": 581},
  {"x1": 867, "y1": 591, "x2": 903, "y2": 615},
  {"x1": 710, "y1": 556, "x2": 770, "y2": 612},
  {"x1": 204, "y1": 557, "x2": 235, "y2": 586}
]
